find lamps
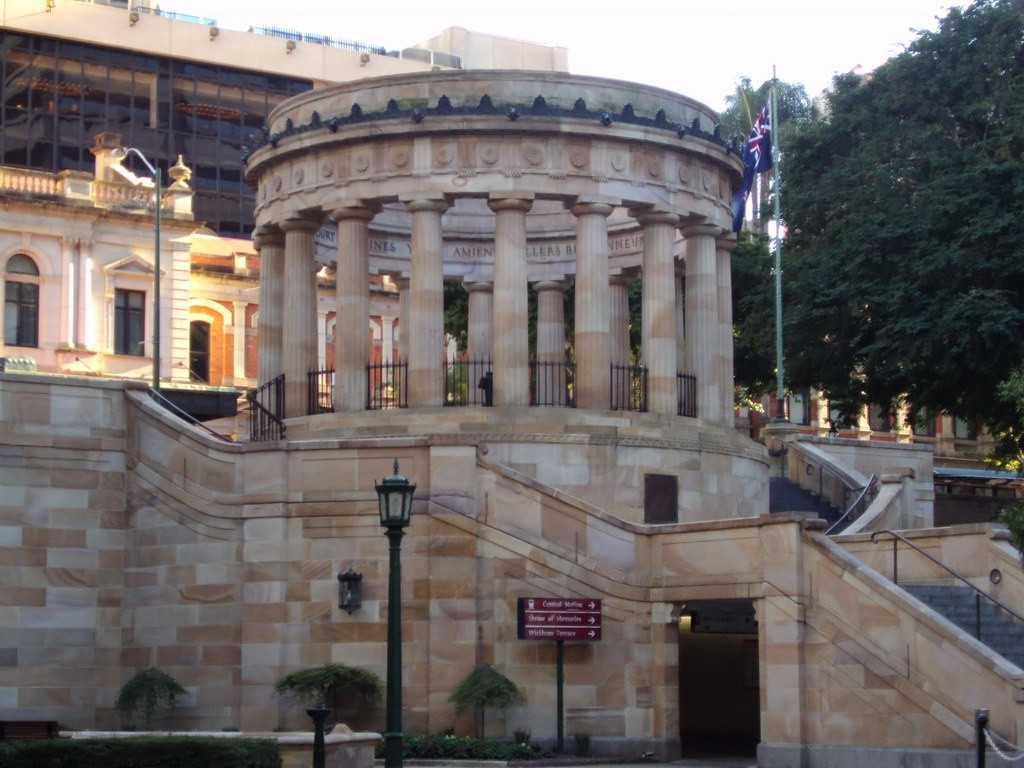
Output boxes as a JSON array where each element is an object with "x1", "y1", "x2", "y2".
[{"x1": 337, "y1": 566, "x2": 362, "y2": 617}]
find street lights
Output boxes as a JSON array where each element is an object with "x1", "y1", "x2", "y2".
[{"x1": 373, "y1": 457, "x2": 418, "y2": 768}]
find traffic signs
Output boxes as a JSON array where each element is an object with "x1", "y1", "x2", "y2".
[{"x1": 518, "y1": 596, "x2": 603, "y2": 642}]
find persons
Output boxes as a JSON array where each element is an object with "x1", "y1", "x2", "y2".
[{"x1": 478, "y1": 372, "x2": 493, "y2": 405}]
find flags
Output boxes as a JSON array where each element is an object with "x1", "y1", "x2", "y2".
[{"x1": 732, "y1": 102, "x2": 773, "y2": 237}]
